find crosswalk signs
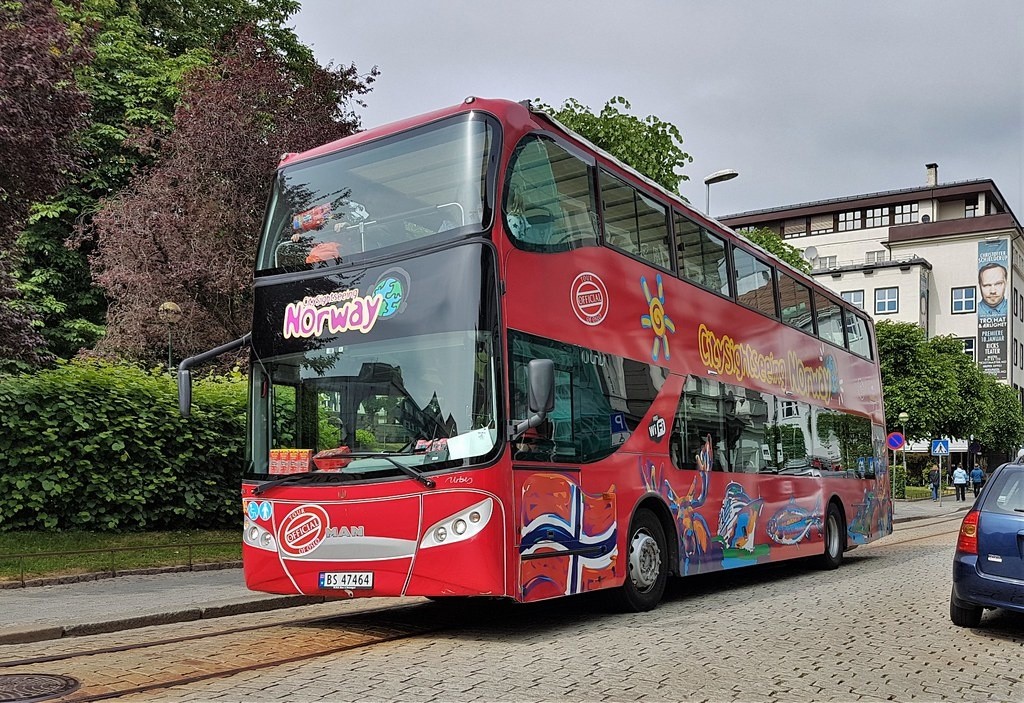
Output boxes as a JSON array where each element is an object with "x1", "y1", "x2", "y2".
[{"x1": 931, "y1": 440, "x2": 950, "y2": 457}]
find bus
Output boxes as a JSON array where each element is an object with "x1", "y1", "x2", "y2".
[{"x1": 175, "y1": 95, "x2": 896, "y2": 614}]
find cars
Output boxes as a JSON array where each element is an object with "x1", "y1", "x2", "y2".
[{"x1": 950, "y1": 454, "x2": 1024, "y2": 628}]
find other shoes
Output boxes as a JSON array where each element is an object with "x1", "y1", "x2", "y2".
[
  {"x1": 934, "y1": 500, "x2": 936, "y2": 501},
  {"x1": 957, "y1": 501, "x2": 960, "y2": 502}
]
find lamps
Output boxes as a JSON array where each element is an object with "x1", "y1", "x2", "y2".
[
  {"x1": 831, "y1": 273, "x2": 841, "y2": 277},
  {"x1": 863, "y1": 270, "x2": 873, "y2": 274},
  {"x1": 899, "y1": 266, "x2": 910, "y2": 270}
]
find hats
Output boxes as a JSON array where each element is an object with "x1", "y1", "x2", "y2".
[{"x1": 930, "y1": 465, "x2": 938, "y2": 470}]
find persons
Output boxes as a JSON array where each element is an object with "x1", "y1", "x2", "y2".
[
  {"x1": 952, "y1": 463, "x2": 969, "y2": 501},
  {"x1": 971, "y1": 463, "x2": 985, "y2": 498},
  {"x1": 927, "y1": 464, "x2": 940, "y2": 501},
  {"x1": 292, "y1": 182, "x2": 531, "y2": 263}
]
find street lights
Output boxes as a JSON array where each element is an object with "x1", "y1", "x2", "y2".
[
  {"x1": 703, "y1": 168, "x2": 740, "y2": 216},
  {"x1": 899, "y1": 411, "x2": 911, "y2": 489}
]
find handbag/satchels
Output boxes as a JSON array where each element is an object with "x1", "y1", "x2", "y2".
[{"x1": 930, "y1": 483, "x2": 933, "y2": 489}]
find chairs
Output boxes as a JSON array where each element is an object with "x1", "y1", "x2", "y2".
[{"x1": 522, "y1": 203, "x2": 555, "y2": 245}]
